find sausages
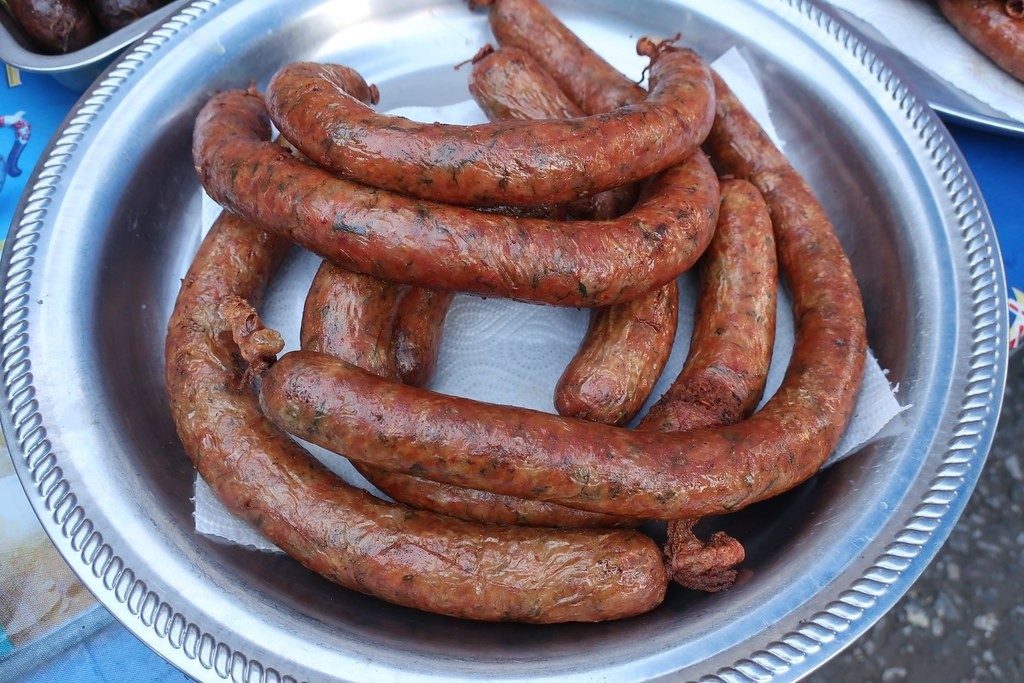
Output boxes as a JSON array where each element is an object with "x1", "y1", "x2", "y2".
[
  {"x1": 4, "y1": 0, "x2": 173, "y2": 53},
  {"x1": 923, "y1": 0, "x2": 1024, "y2": 80},
  {"x1": 162, "y1": 0, "x2": 866, "y2": 626}
]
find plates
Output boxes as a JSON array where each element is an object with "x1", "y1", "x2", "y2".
[{"x1": 0, "y1": 0, "x2": 1024, "y2": 683}]
